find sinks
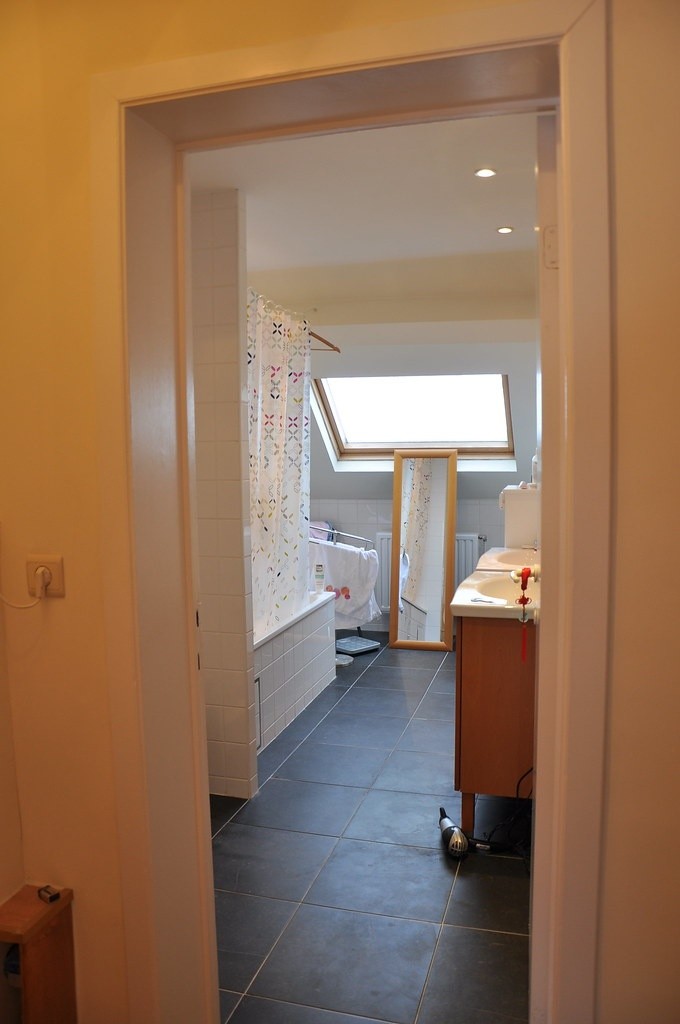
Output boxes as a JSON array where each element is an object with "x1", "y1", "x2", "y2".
[
  {"x1": 475, "y1": 542, "x2": 542, "y2": 577},
  {"x1": 448, "y1": 571, "x2": 544, "y2": 625}
]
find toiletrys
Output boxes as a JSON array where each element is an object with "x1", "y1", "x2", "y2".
[{"x1": 531, "y1": 446, "x2": 539, "y2": 485}]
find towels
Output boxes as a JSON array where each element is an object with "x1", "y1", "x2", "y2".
[
  {"x1": 308, "y1": 538, "x2": 383, "y2": 631},
  {"x1": 399, "y1": 551, "x2": 412, "y2": 614}
]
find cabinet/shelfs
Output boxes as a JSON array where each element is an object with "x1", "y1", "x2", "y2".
[
  {"x1": 444, "y1": 569, "x2": 536, "y2": 842},
  {"x1": 398, "y1": 594, "x2": 428, "y2": 640}
]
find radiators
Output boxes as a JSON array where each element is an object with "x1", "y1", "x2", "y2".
[{"x1": 376, "y1": 531, "x2": 481, "y2": 615}]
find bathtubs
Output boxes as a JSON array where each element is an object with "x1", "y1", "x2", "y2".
[{"x1": 252, "y1": 587, "x2": 338, "y2": 758}]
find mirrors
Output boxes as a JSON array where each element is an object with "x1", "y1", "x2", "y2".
[{"x1": 387, "y1": 448, "x2": 456, "y2": 651}]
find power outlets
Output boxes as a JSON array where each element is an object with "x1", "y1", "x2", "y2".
[{"x1": 26, "y1": 554, "x2": 65, "y2": 598}]
